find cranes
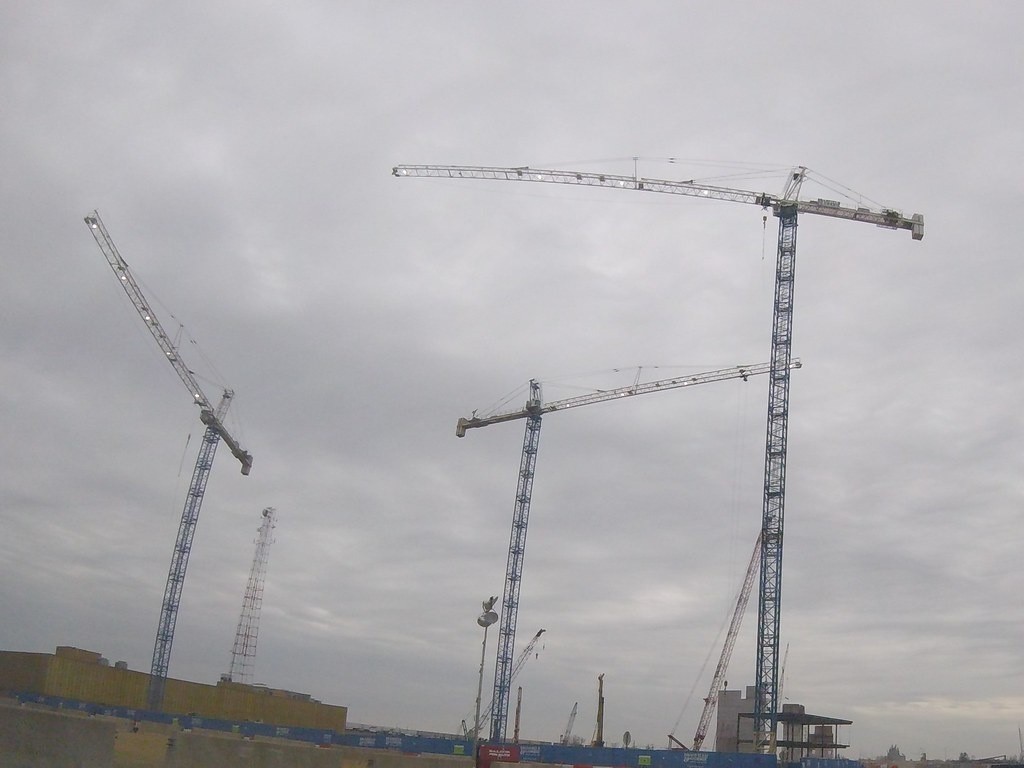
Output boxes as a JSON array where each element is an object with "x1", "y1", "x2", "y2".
[
  {"x1": 85, "y1": 206, "x2": 253, "y2": 718},
  {"x1": 392, "y1": 162, "x2": 925, "y2": 758},
  {"x1": 454, "y1": 358, "x2": 805, "y2": 741}
]
